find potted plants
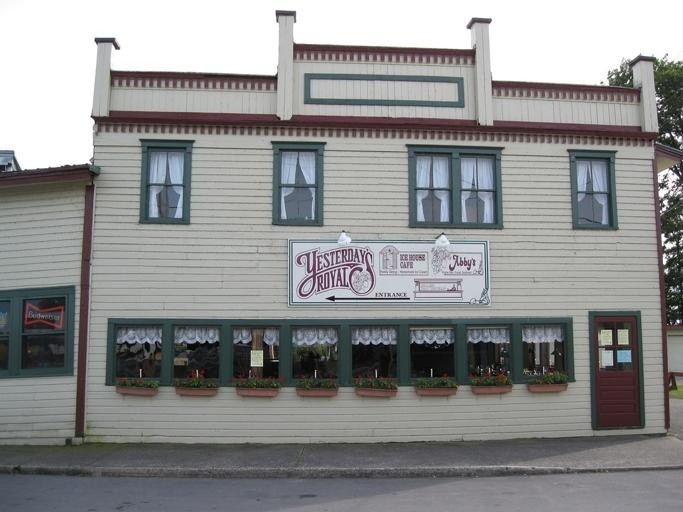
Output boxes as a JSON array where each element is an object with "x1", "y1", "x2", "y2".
[
  {"x1": 528, "y1": 372, "x2": 568, "y2": 392},
  {"x1": 296, "y1": 379, "x2": 338, "y2": 397},
  {"x1": 173, "y1": 378, "x2": 219, "y2": 395},
  {"x1": 115, "y1": 379, "x2": 160, "y2": 397},
  {"x1": 356, "y1": 378, "x2": 399, "y2": 398},
  {"x1": 235, "y1": 379, "x2": 281, "y2": 398},
  {"x1": 415, "y1": 378, "x2": 457, "y2": 395},
  {"x1": 470, "y1": 372, "x2": 512, "y2": 394}
]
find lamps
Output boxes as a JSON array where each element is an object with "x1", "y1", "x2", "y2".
[
  {"x1": 434, "y1": 233, "x2": 451, "y2": 248},
  {"x1": 337, "y1": 230, "x2": 352, "y2": 246}
]
